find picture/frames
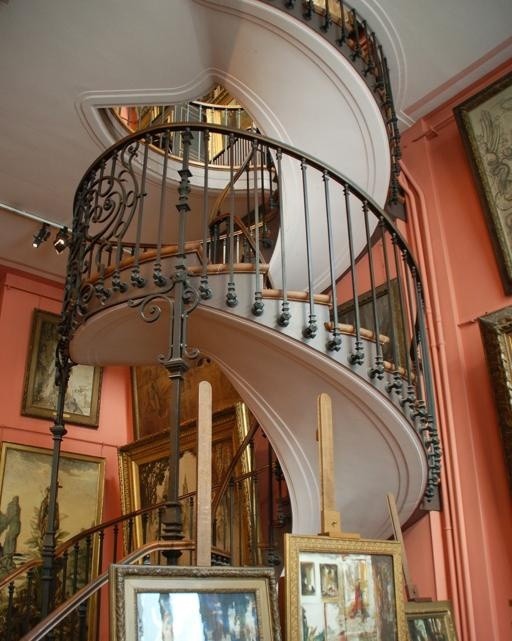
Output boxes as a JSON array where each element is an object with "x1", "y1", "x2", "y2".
[
  {"x1": 404, "y1": 600, "x2": 459, "y2": 641},
  {"x1": 477, "y1": 304, "x2": 512, "y2": 498},
  {"x1": 330, "y1": 275, "x2": 412, "y2": 400},
  {"x1": 285, "y1": 535, "x2": 410, "y2": 641},
  {"x1": 130, "y1": 354, "x2": 244, "y2": 440},
  {"x1": 453, "y1": 68, "x2": 512, "y2": 295},
  {"x1": 21, "y1": 307, "x2": 105, "y2": 429},
  {"x1": 116, "y1": 401, "x2": 261, "y2": 568},
  {"x1": 0, "y1": 441, "x2": 107, "y2": 641},
  {"x1": 108, "y1": 563, "x2": 282, "y2": 641}
]
